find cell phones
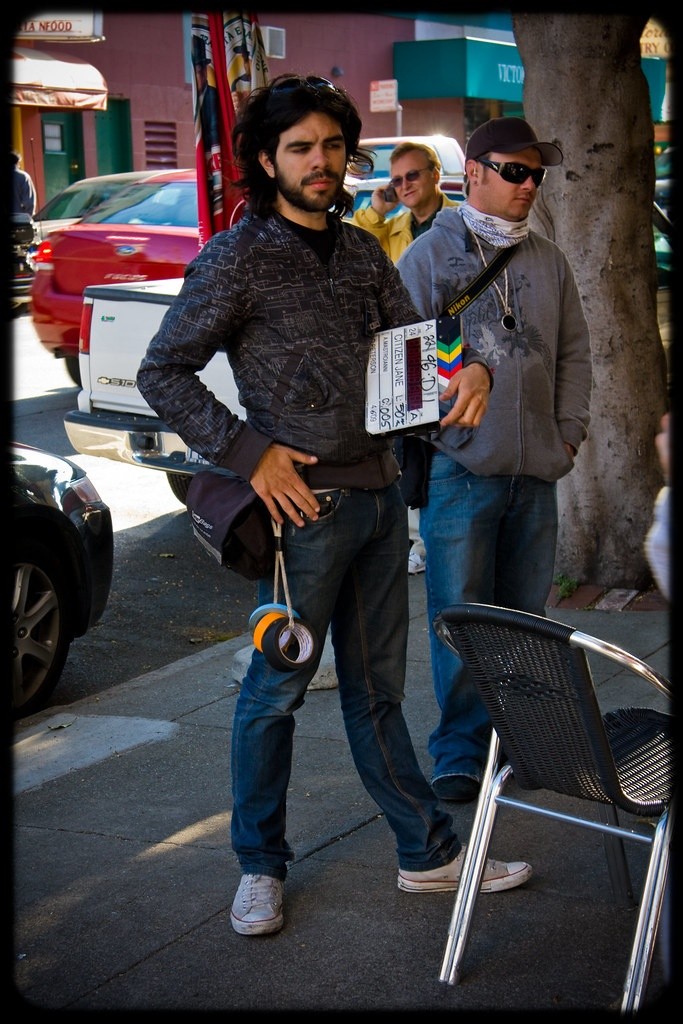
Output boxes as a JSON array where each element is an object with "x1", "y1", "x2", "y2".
[{"x1": 383, "y1": 187, "x2": 398, "y2": 202}]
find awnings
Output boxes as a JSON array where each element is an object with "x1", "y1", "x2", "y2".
[{"x1": 13, "y1": 47, "x2": 109, "y2": 111}]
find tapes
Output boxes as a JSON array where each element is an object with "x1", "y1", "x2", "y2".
[
  {"x1": 253, "y1": 613, "x2": 288, "y2": 654},
  {"x1": 262, "y1": 618, "x2": 320, "y2": 673},
  {"x1": 248, "y1": 603, "x2": 300, "y2": 639}
]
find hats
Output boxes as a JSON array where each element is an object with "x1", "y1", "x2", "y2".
[{"x1": 465, "y1": 117, "x2": 564, "y2": 171}]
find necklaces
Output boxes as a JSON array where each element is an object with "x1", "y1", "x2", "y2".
[{"x1": 471, "y1": 231, "x2": 517, "y2": 331}]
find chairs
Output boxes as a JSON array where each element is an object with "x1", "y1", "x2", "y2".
[{"x1": 432, "y1": 603, "x2": 671, "y2": 1014}]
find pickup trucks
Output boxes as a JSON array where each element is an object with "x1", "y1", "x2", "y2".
[{"x1": 60, "y1": 274, "x2": 248, "y2": 509}]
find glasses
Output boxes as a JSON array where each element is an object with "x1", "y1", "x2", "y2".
[
  {"x1": 390, "y1": 167, "x2": 433, "y2": 186},
  {"x1": 271, "y1": 75, "x2": 338, "y2": 94},
  {"x1": 475, "y1": 158, "x2": 547, "y2": 187}
]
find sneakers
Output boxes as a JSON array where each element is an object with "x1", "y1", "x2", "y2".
[
  {"x1": 230, "y1": 873, "x2": 284, "y2": 935},
  {"x1": 397, "y1": 845, "x2": 533, "y2": 893}
]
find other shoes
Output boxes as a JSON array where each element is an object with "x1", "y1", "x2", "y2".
[{"x1": 432, "y1": 775, "x2": 480, "y2": 801}]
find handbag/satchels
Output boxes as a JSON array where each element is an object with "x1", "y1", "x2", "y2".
[
  {"x1": 394, "y1": 432, "x2": 428, "y2": 510},
  {"x1": 185, "y1": 470, "x2": 274, "y2": 580}
]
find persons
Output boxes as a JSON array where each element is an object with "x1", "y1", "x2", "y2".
[
  {"x1": 396, "y1": 119, "x2": 592, "y2": 801},
  {"x1": 644, "y1": 414, "x2": 670, "y2": 598},
  {"x1": 136, "y1": 73, "x2": 533, "y2": 935},
  {"x1": 353, "y1": 143, "x2": 463, "y2": 573},
  {"x1": 14, "y1": 153, "x2": 39, "y2": 216}
]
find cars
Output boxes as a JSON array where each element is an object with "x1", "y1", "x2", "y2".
[
  {"x1": 24, "y1": 168, "x2": 195, "y2": 273},
  {"x1": 6, "y1": 439, "x2": 115, "y2": 723},
  {"x1": 343, "y1": 129, "x2": 465, "y2": 222},
  {"x1": 650, "y1": 177, "x2": 669, "y2": 350},
  {"x1": 29, "y1": 168, "x2": 200, "y2": 390}
]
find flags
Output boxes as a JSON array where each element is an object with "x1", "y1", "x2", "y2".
[{"x1": 191, "y1": 14, "x2": 270, "y2": 250}]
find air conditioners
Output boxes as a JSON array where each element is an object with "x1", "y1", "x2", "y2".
[{"x1": 259, "y1": 26, "x2": 286, "y2": 59}]
